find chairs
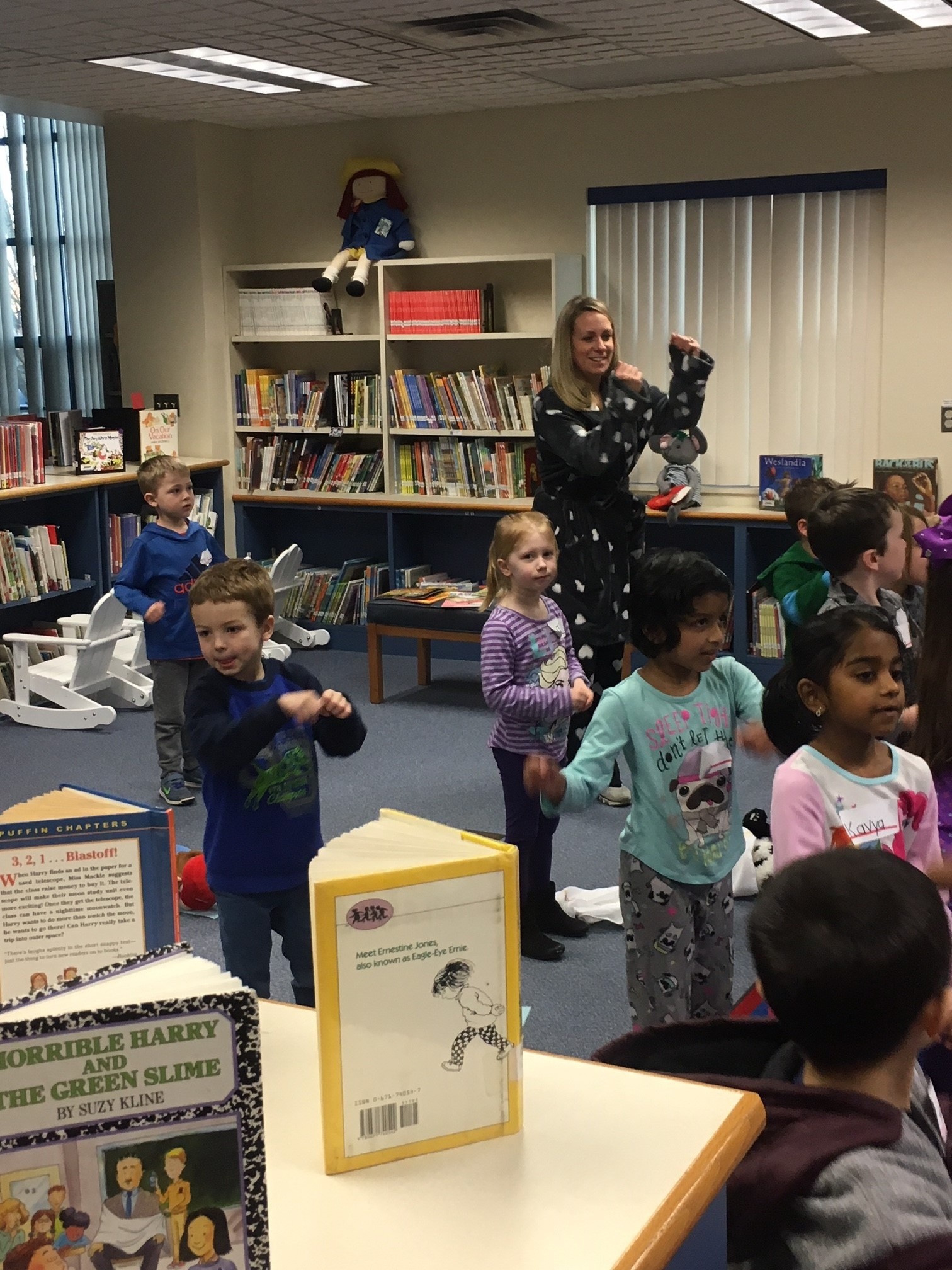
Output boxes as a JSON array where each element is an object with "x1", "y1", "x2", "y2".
[
  {"x1": 262, "y1": 543, "x2": 329, "y2": 662},
  {"x1": 0, "y1": 588, "x2": 158, "y2": 729}
]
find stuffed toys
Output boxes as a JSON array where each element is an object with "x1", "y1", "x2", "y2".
[
  {"x1": 175, "y1": 844, "x2": 217, "y2": 911},
  {"x1": 312, "y1": 156, "x2": 415, "y2": 296},
  {"x1": 635, "y1": 427, "x2": 707, "y2": 527}
]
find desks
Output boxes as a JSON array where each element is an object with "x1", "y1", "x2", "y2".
[{"x1": 0, "y1": 1002, "x2": 766, "y2": 1270}]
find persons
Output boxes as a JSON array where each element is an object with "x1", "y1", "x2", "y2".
[
  {"x1": 182, "y1": 556, "x2": 369, "y2": 1006},
  {"x1": 478, "y1": 511, "x2": 592, "y2": 960},
  {"x1": 527, "y1": 296, "x2": 714, "y2": 805},
  {"x1": 757, "y1": 473, "x2": 952, "y2": 852},
  {"x1": 113, "y1": 455, "x2": 231, "y2": 805},
  {"x1": 583, "y1": 847, "x2": 952, "y2": 1270},
  {"x1": 759, "y1": 604, "x2": 952, "y2": 1093},
  {"x1": 878, "y1": 470, "x2": 935, "y2": 517},
  {"x1": 521, "y1": 549, "x2": 773, "y2": 1031}
]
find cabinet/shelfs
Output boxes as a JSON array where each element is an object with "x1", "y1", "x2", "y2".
[
  {"x1": 621, "y1": 495, "x2": 807, "y2": 682},
  {"x1": 0, "y1": 458, "x2": 229, "y2": 647},
  {"x1": 231, "y1": 252, "x2": 557, "y2": 662}
]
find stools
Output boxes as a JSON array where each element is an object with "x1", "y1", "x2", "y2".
[{"x1": 368, "y1": 599, "x2": 492, "y2": 702}]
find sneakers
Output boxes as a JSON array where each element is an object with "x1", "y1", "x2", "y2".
[
  {"x1": 598, "y1": 784, "x2": 631, "y2": 807},
  {"x1": 159, "y1": 779, "x2": 195, "y2": 805},
  {"x1": 183, "y1": 765, "x2": 204, "y2": 789}
]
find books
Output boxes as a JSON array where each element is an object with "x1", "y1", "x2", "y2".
[
  {"x1": 0, "y1": 408, "x2": 219, "y2": 704},
  {"x1": 308, "y1": 808, "x2": 526, "y2": 1174},
  {"x1": 720, "y1": 581, "x2": 784, "y2": 659},
  {"x1": 234, "y1": 286, "x2": 553, "y2": 628},
  {"x1": 758, "y1": 454, "x2": 823, "y2": 512},
  {"x1": 0, "y1": 781, "x2": 179, "y2": 1012},
  {"x1": 872, "y1": 458, "x2": 938, "y2": 528},
  {"x1": 0, "y1": 941, "x2": 271, "y2": 1270}
]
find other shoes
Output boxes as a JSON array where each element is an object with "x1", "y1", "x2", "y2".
[
  {"x1": 519, "y1": 929, "x2": 566, "y2": 960},
  {"x1": 540, "y1": 906, "x2": 589, "y2": 938}
]
